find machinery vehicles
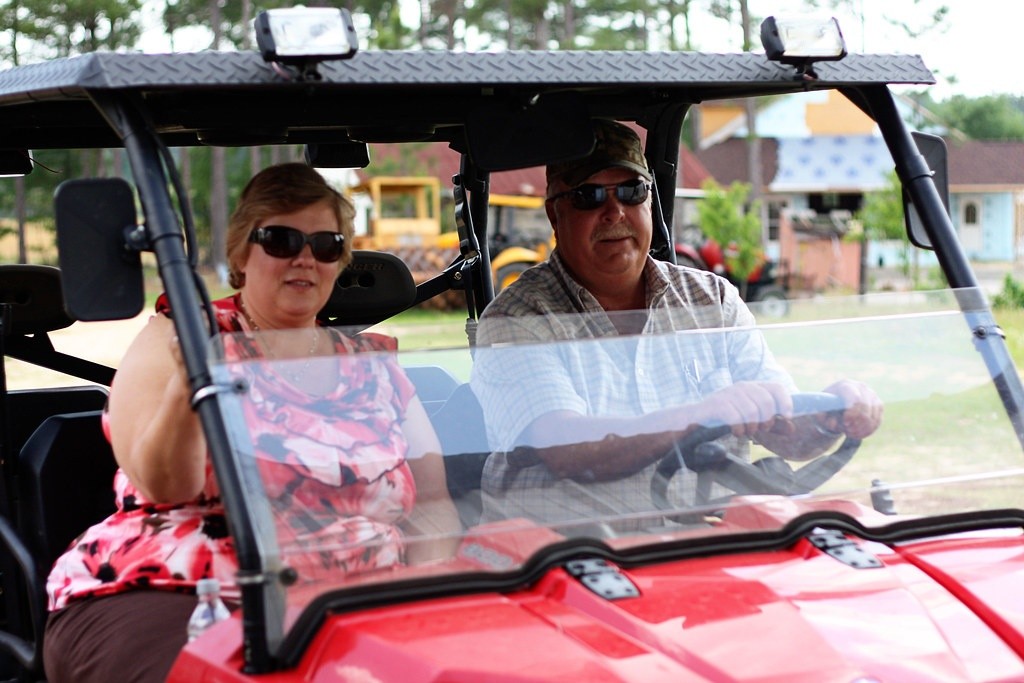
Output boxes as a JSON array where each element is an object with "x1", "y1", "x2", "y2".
[
  {"x1": 344, "y1": 175, "x2": 560, "y2": 320},
  {"x1": 647, "y1": 184, "x2": 792, "y2": 324}
]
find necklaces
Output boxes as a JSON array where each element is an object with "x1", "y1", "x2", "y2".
[{"x1": 241, "y1": 304, "x2": 320, "y2": 379}]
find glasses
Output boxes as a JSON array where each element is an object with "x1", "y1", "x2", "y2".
[
  {"x1": 249, "y1": 225, "x2": 346, "y2": 264},
  {"x1": 546, "y1": 180, "x2": 653, "y2": 212}
]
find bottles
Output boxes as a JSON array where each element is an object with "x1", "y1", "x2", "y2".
[{"x1": 187, "y1": 579, "x2": 231, "y2": 644}]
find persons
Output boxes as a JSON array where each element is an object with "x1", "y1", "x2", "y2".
[
  {"x1": 470, "y1": 117, "x2": 884, "y2": 542},
  {"x1": 46, "y1": 161, "x2": 457, "y2": 682}
]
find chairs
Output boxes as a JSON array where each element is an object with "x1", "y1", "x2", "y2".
[
  {"x1": 310, "y1": 251, "x2": 495, "y2": 498},
  {"x1": 0, "y1": 262, "x2": 123, "y2": 619}
]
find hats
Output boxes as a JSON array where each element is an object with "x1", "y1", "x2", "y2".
[{"x1": 546, "y1": 118, "x2": 654, "y2": 189}]
